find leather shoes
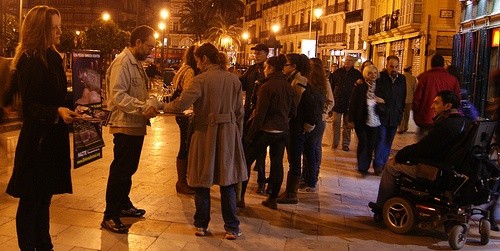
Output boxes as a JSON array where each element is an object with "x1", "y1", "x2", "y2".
[
  {"x1": 120, "y1": 205, "x2": 145, "y2": 217},
  {"x1": 100, "y1": 217, "x2": 128, "y2": 233}
]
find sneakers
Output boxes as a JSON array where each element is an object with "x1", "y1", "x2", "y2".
[
  {"x1": 277, "y1": 190, "x2": 298, "y2": 204},
  {"x1": 297, "y1": 181, "x2": 318, "y2": 193},
  {"x1": 262, "y1": 195, "x2": 277, "y2": 209},
  {"x1": 195, "y1": 227, "x2": 211, "y2": 236},
  {"x1": 225, "y1": 230, "x2": 242, "y2": 239}
]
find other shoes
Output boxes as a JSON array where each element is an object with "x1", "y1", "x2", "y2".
[
  {"x1": 256, "y1": 182, "x2": 265, "y2": 194},
  {"x1": 236, "y1": 199, "x2": 245, "y2": 208},
  {"x1": 267, "y1": 184, "x2": 272, "y2": 194},
  {"x1": 331, "y1": 130, "x2": 406, "y2": 176}
]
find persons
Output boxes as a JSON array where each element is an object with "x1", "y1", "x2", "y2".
[
  {"x1": 4, "y1": 6, "x2": 93, "y2": 251},
  {"x1": 98, "y1": 26, "x2": 162, "y2": 235},
  {"x1": 366, "y1": 90, "x2": 472, "y2": 218},
  {"x1": 336, "y1": 53, "x2": 459, "y2": 173},
  {"x1": 164, "y1": 42, "x2": 335, "y2": 239}
]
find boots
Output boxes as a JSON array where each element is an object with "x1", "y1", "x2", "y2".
[{"x1": 176, "y1": 157, "x2": 195, "y2": 194}]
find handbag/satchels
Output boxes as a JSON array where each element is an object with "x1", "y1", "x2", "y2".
[{"x1": 170, "y1": 89, "x2": 182, "y2": 101}]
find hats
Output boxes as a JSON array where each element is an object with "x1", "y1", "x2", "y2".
[{"x1": 251, "y1": 43, "x2": 269, "y2": 53}]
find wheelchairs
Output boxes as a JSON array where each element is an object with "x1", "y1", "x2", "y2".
[{"x1": 372, "y1": 116, "x2": 500, "y2": 250}]
